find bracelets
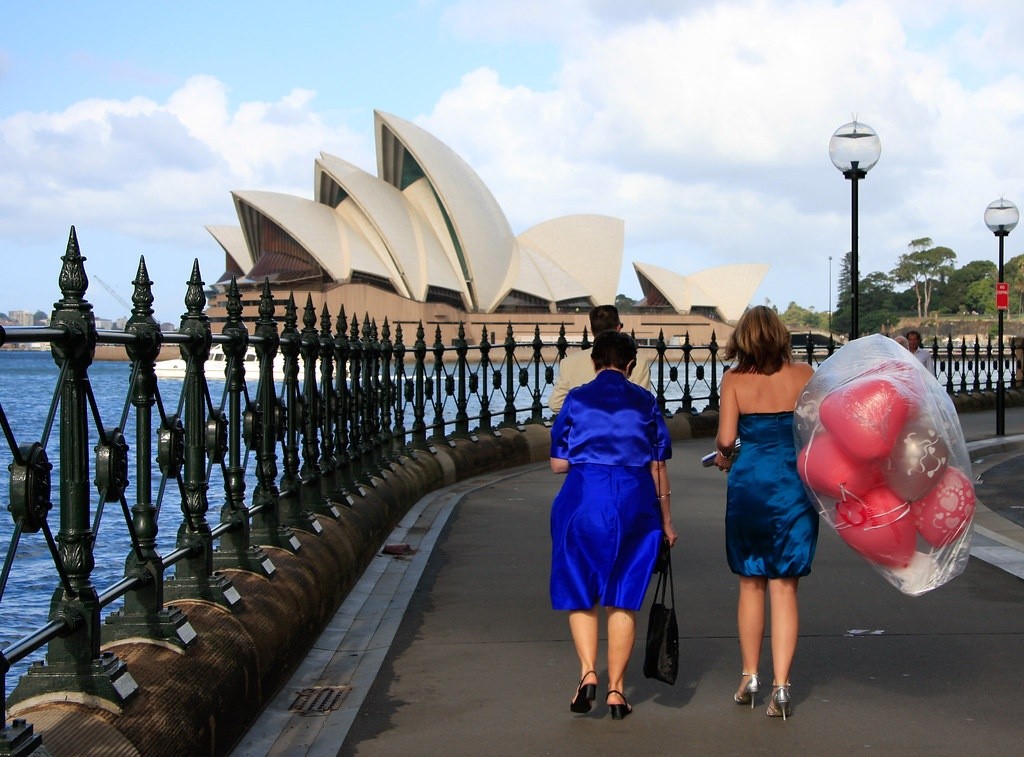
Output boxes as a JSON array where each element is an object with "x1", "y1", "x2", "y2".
[
  {"x1": 719, "y1": 450, "x2": 734, "y2": 460},
  {"x1": 658, "y1": 490, "x2": 672, "y2": 500}
]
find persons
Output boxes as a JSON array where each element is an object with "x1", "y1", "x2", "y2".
[
  {"x1": 549, "y1": 305, "x2": 651, "y2": 415},
  {"x1": 549, "y1": 331, "x2": 679, "y2": 721},
  {"x1": 895, "y1": 330, "x2": 934, "y2": 376},
  {"x1": 715, "y1": 305, "x2": 819, "y2": 721}
]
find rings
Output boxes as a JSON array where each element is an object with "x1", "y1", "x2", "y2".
[
  {"x1": 674, "y1": 543, "x2": 675, "y2": 544},
  {"x1": 723, "y1": 469, "x2": 727, "y2": 472}
]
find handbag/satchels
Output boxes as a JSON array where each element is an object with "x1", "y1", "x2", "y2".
[{"x1": 642, "y1": 540, "x2": 679, "y2": 686}]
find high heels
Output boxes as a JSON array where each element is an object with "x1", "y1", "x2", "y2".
[
  {"x1": 734, "y1": 672, "x2": 761, "y2": 708},
  {"x1": 766, "y1": 683, "x2": 791, "y2": 721},
  {"x1": 606, "y1": 690, "x2": 631, "y2": 720},
  {"x1": 570, "y1": 670, "x2": 598, "y2": 713}
]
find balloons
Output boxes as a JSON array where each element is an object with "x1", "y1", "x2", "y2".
[{"x1": 798, "y1": 358, "x2": 976, "y2": 569}]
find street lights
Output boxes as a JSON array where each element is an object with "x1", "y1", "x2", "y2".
[
  {"x1": 984, "y1": 196, "x2": 1020, "y2": 436},
  {"x1": 829, "y1": 117, "x2": 879, "y2": 334}
]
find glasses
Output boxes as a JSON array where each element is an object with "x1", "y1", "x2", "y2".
[{"x1": 618, "y1": 321, "x2": 623, "y2": 329}]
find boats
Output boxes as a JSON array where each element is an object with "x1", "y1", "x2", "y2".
[{"x1": 153, "y1": 344, "x2": 320, "y2": 375}]
